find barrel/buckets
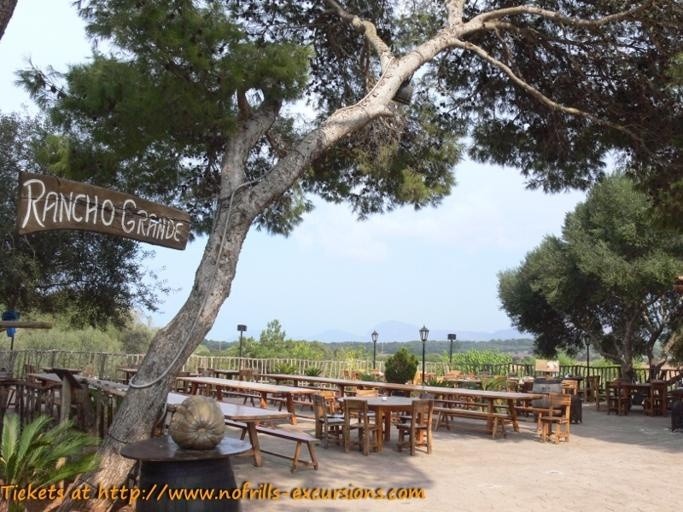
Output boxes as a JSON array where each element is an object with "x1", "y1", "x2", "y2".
[{"x1": 532, "y1": 378, "x2": 564, "y2": 423}]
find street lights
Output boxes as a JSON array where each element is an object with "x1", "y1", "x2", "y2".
[
  {"x1": 585, "y1": 335, "x2": 591, "y2": 401},
  {"x1": 419, "y1": 324, "x2": 429, "y2": 382},
  {"x1": 371, "y1": 330, "x2": 379, "y2": 369}
]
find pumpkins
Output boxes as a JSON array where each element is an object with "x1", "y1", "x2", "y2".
[{"x1": 168, "y1": 396, "x2": 228, "y2": 452}]
